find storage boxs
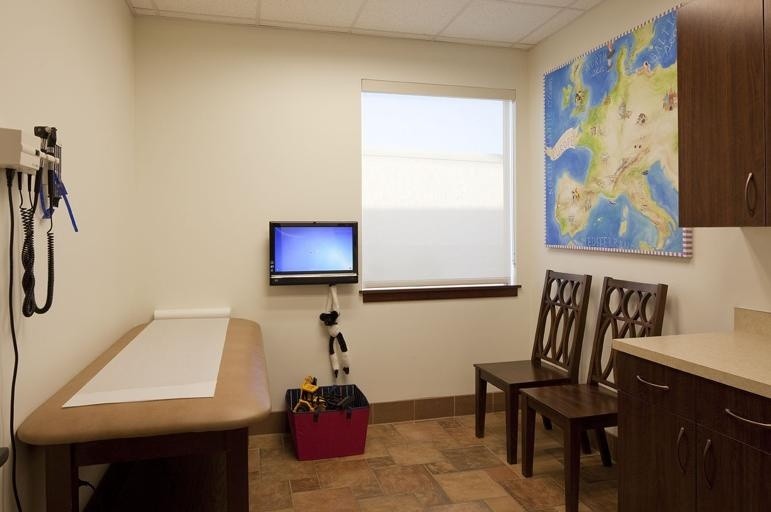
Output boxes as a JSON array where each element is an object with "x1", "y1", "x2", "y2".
[{"x1": 284, "y1": 383, "x2": 372, "y2": 462}]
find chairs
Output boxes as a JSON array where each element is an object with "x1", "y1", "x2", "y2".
[
  {"x1": 515, "y1": 276, "x2": 668, "y2": 512},
  {"x1": 468, "y1": 269, "x2": 592, "y2": 464}
]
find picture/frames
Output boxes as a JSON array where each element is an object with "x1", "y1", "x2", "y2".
[{"x1": 542, "y1": 2, "x2": 693, "y2": 260}]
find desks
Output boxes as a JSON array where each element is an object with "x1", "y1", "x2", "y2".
[{"x1": 16, "y1": 317, "x2": 270, "y2": 512}]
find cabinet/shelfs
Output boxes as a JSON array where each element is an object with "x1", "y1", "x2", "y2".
[
  {"x1": 676, "y1": 0, "x2": 771, "y2": 229},
  {"x1": 611, "y1": 349, "x2": 771, "y2": 512}
]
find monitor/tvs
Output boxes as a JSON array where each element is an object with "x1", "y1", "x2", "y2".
[{"x1": 268, "y1": 221, "x2": 358, "y2": 285}]
